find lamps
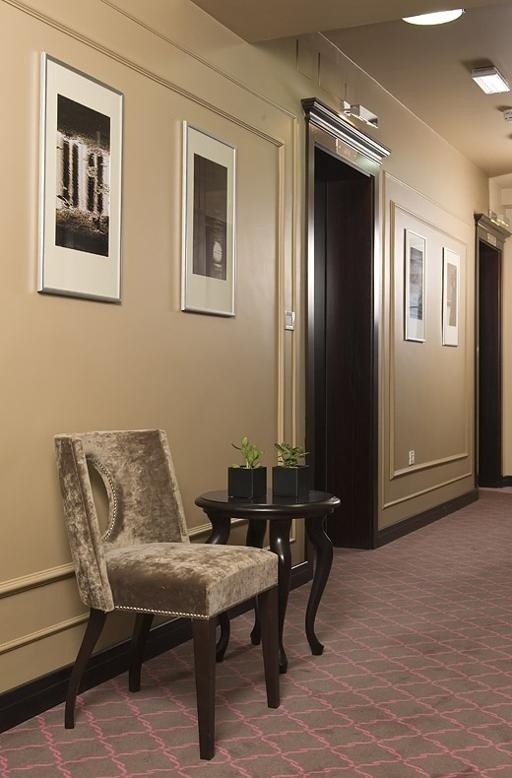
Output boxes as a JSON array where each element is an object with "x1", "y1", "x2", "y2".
[
  {"x1": 344, "y1": 100, "x2": 378, "y2": 128},
  {"x1": 470, "y1": 66, "x2": 512, "y2": 96},
  {"x1": 488, "y1": 209, "x2": 509, "y2": 227}
]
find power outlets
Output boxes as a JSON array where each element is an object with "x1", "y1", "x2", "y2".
[{"x1": 408, "y1": 450, "x2": 415, "y2": 465}]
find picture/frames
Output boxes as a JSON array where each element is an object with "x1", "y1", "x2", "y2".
[
  {"x1": 404, "y1": 227, "x2": 427, "y2": 343},
  {"x1": 36, "y1": 50, "x2": 125, "y2": 304},
  {"x1": 442, "y1": 246, "x2": 461, "y2": 346},
  {"x1": 181, "y1": 119, "x2": 237, "y2": 317}
]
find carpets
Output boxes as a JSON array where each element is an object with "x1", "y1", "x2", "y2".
[{"x1": 0, "y1": 489, "x2": 511, "y2": 778}]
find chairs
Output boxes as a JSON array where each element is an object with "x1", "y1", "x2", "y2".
[{"x1": 54, "y1": 428, "x2": 281, "y2": 760}]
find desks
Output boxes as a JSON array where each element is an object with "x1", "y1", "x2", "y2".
[{"x1": 194, "y1": 490, "x2": 341, "y2": 674}]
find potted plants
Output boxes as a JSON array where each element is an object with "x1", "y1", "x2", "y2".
[
  {"x1": 228, "y1": 434, "x2": 267, "y2": 498},
  {"x1": 272, "y1": 441, "x2": 310, "y2": 498}
]
